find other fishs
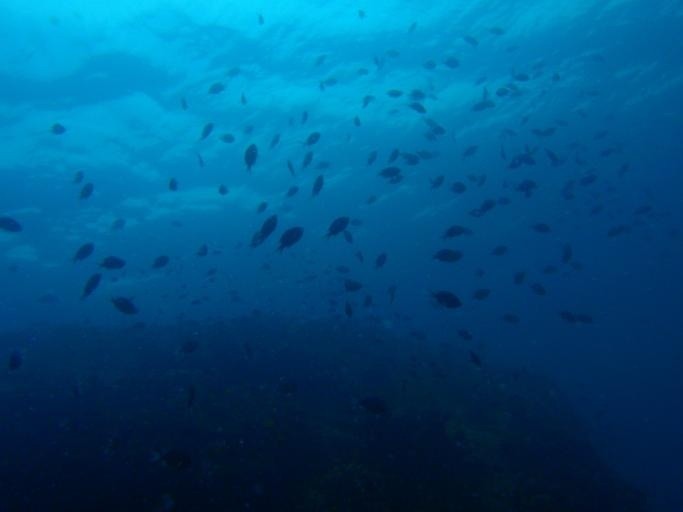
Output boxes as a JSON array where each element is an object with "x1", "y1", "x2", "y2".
[{"x1": 47, "y1": 8, "x2": 655, "y2": 512}]
